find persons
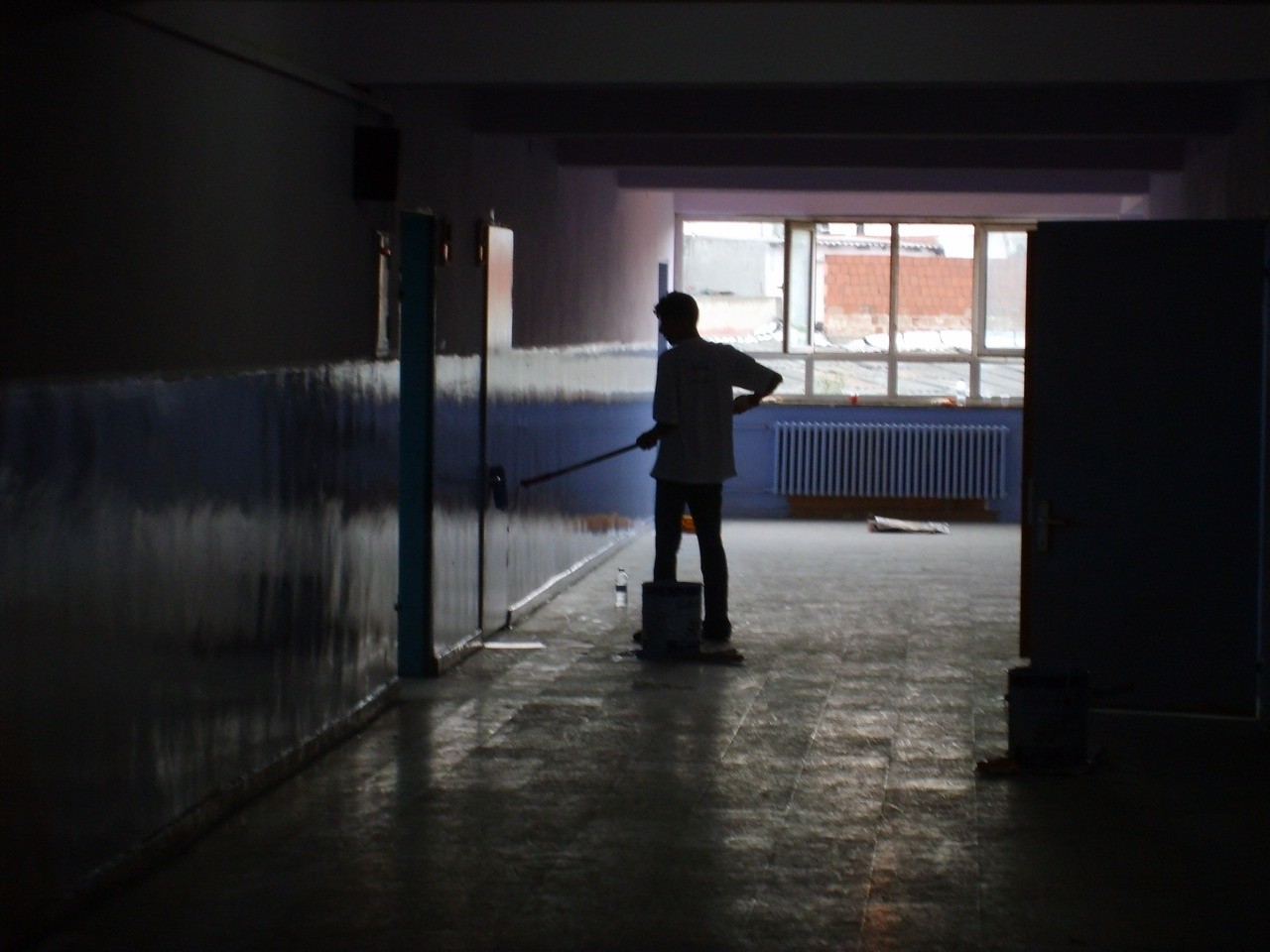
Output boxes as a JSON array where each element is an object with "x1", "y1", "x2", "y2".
[{"x1": 635, "y1": 290, "x2": 784, "y2": 642}]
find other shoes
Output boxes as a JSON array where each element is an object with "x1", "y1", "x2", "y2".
[
  {"x1": 702, "y1": 624, "x2": 734, "y2": 642},
  {"x1": 634, "y1": 631, "x2": 642, "y2": 641}
]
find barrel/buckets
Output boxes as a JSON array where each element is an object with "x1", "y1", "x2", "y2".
[{"x1": 642, "y1": 581, "x2": 703, "y2": 643}]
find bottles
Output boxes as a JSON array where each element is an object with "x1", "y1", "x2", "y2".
[{"x1": 615, "y1": 568, "x2": 628, "y2": 608}]
